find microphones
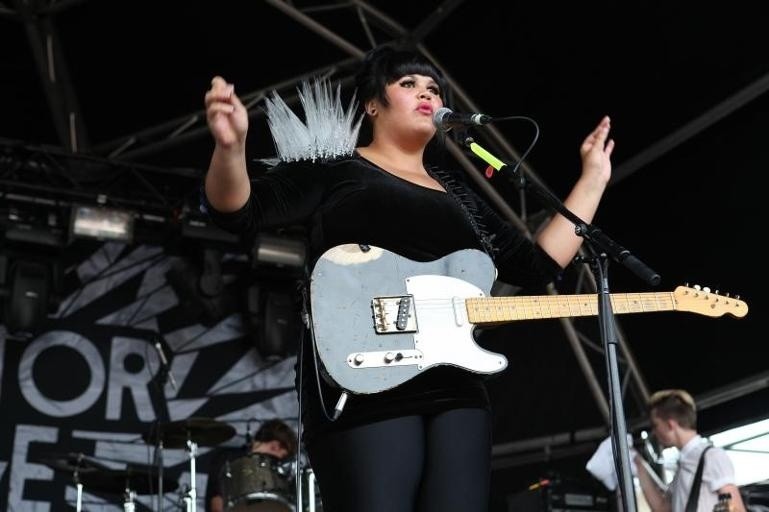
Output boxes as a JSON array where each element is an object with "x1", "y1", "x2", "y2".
[
  {"x1": 150, "y1": 336, "x2": 177, "y2": 390},
  {"x1": 432, "y1": 108, "x2": 488, "y2": 132}
]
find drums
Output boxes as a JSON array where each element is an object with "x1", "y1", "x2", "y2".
[{"x1": 224, "y1": 453, "x2": 297, "y2": 510}]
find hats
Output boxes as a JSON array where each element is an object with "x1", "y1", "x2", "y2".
[{"x1": 261, "y1": 418, "x2": 299, "y2": 458}]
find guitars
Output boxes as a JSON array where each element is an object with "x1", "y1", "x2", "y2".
[{"x1": 310, "y1": 244, "x2": 750, "y2": 394}]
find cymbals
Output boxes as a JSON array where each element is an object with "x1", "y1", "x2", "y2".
[
  {"x1": 44, "y1": 451, "x2": 110, "y2": 475},
  {"x1": 142, "y1": 417, "x2": 236, "y2": 450}
]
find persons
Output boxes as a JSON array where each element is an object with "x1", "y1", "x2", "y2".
[
  {"x1": 634, "y1": 390, "x2": 746, "y2": 512},
  {"x1": 202, "y1": 41, "x2": 616, "y2": 512},
  {"x1": 204, "y1": 418, "x2": 299, "y2": 512}
]
